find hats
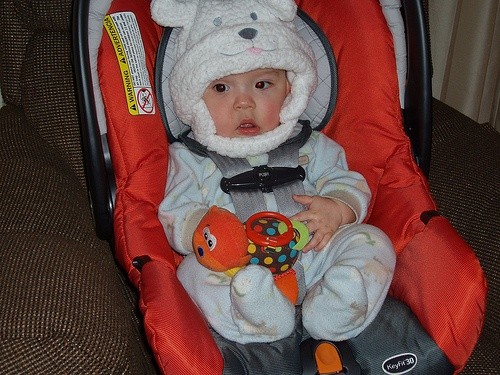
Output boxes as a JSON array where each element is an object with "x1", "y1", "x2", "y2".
[{"x1": 150, "y1": 0, "x2": 320, "y2": 158}]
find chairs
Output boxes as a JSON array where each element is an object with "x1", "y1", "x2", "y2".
[{"x1": 71, "y1": 0, "x2": 489, "y2": 374}]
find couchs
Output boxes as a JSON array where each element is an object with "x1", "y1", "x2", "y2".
[{"x1": 2, "y1": 1, "x2": 499, "y2": 374}]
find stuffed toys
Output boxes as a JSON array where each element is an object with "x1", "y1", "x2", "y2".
[{"x1": 193, "y1": 205, "x2": 311, "y2": 306}]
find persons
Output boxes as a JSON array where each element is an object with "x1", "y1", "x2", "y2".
[{"x1": 150, "y1": 0, "x2": 397, "y2": 345}]
